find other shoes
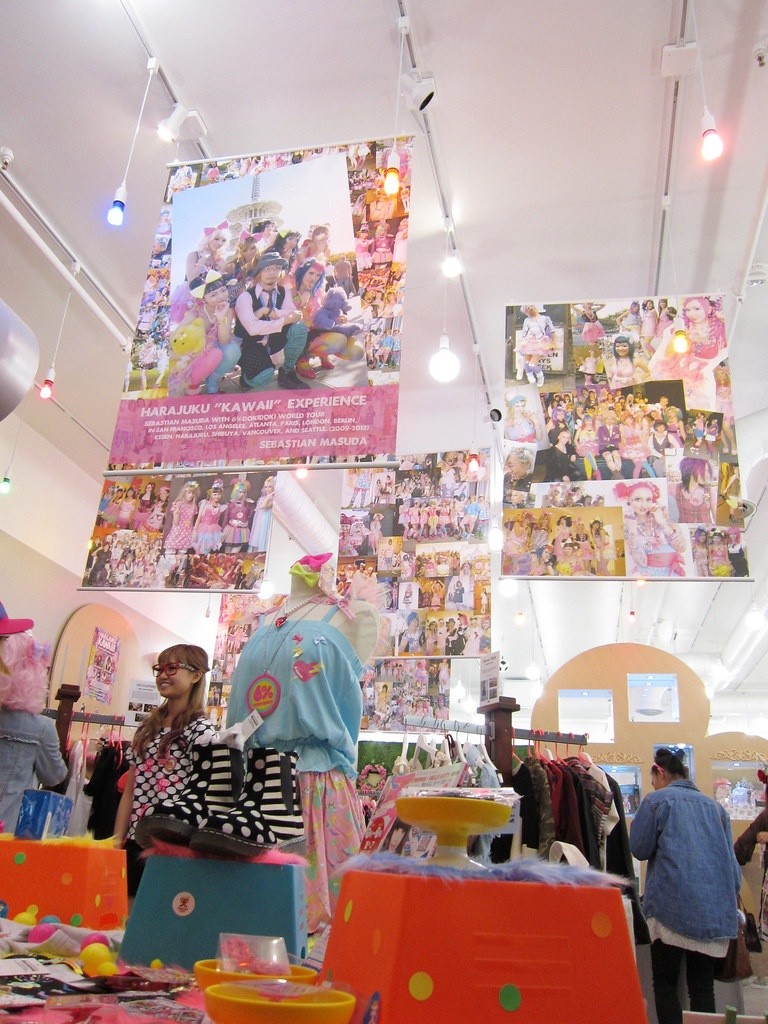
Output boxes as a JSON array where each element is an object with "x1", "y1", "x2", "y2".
[
  {"x1": 723, "y1": 448, "x2": 728, "y2": 454},
  {"x1": 205, "y1": 379, "x2": 219, "y2": 394},
  {"x1": 186, "y1": 385, "x2": 201, "y2": 395},
  {"x1": 690, "y1": 447, "x2": 700, "y2": 455},
  {"x1": 402, "y1": 533, "x2": 473, "y2": 542},
  {"x1": 731, "y1": 449, "x2": 737, "y2": 455}
]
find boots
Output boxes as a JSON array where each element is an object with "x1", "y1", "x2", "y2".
[
  {"x1": 142, "y1": 744, "x2": 247, "y2": 846},
  {"x1": 189, "y1": 747, "x2": 307, "y2": 857},
  {"x1": 526, "y1": 371, "x2": 536, "y2": 384},
  {"x1": 613, "y1": 470, "x2": 625, "y2": 480},
  {"x1": 240, "y1": 376, "x2": 251, "y2": 391},
  {"x1": 277, "y1": 367, "x2": 310, "y2": 389},
  {"x1": 535, "y1": 371, "x2": 545, "y2": 387}
]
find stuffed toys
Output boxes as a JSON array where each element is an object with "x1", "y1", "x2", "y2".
[{"x1": 314, "y1": 288, "x2": 362, "y2": 336}]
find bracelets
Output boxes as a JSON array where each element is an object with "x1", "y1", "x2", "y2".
[
  {"x1": 196, "y1": 263, "x2": 202, "y2": 267},
  {"x1": 175, "y1": 511, "x2": 178, "y2": 514},
  {"x1": 218, "y1": 316, "x2": 227, "y2": 324},
  {"x1": 267, "y1": 309, "x2": 272, "y2": 315}
]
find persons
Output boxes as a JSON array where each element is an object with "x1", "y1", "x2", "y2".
[
  {"x1": 380, "y1": 816, "x2": 412, "y2": 856},
  {"x1": 222, "y1": 553, "x2": 367, "y2": 934},
  {"x1": 501, "y1": 297, "x2": 750, "y2": 579},
  {"x1": 83, "y1": 476, "x2": 275, "y2": 591},
  {"x1": 734, "y1": 765, "x2": 768, "y2": 943},
  {"x1": 0, "y1": 602, "x2": 67, "y2": 833},
  {"x1": 113, "y1": 644, "x2": 216, "y2": 898},
  {"x1": 630, "y1": 749, "x2": 742, "y2": 1024},
  {"x1": 130, "y1": 138, "x2": 411, "y2": 395},
  {"x1": 339, "y1": 452, "x2": 489, "y2": 733}
]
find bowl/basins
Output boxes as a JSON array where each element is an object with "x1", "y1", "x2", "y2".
[
  {"x1": 205, "y1": 982, "x2": 356, "y2": 1024},
  {"x1": 193, "y1": 959, "x2": 317, "y2": 989}
]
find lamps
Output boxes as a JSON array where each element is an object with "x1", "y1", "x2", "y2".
[
  {"x1": 483, "y1": 409, "x2": 502, "y2": 423},
  {"x1": 400, "y1": 70, "x2": 434, "y2": 110},
  {"x1": 156, "y1": 105, "x2": 190, "y2": 144}
]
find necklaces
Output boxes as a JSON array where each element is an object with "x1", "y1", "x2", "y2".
[
  {"x1": 204, "y1": 304, "x2": 217, "y2": 322},
  {"x1": 637, "y1": 516, "x2": 655, "y2": 542},
  {"x1": 688, "y1": 485, "x2": 698, "y2": 502},
  {"x1": 276, "y1": 593, "x2": 325, "y2": 628}
]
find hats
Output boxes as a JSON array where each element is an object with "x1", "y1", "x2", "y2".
[
  {"x1": 0, "y1": 602, "x2": 34, "y2": 635},
  {"x1": 653, "y1": 420, "x2": 668, "y2": 431},
  {"x1": 249, "y1": 252, "x2": 289, "y2": 275},
  {"x1": 158, "y1": 486, "x2": 169, "y2": 496}
]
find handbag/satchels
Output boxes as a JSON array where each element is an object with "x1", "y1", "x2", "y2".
[{"x1": 710, "y1": 912, "x2": 753, "y2": 982}]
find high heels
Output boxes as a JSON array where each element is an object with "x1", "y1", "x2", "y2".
[
  {"x1": 321, "y1": 358, "x2": 335, "y2": 368},
  {"x1": 296, "y1": 357, "x2": 316, "y2": 378}
]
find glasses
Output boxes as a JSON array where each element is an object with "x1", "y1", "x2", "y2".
[
  {"x1": 152, "y1": 662, "x2": 197, "y2": 677},
  {"x1": 261, "y1": 267, "x2": 282, "y2": 271}
]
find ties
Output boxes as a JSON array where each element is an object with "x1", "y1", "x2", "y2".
[{"x1": 263, "y1": 288, "x2": 276, "y2": 308}]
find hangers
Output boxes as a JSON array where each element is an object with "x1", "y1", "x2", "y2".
[
  {"x1": 45, "y1": 709, "x2": 125, "y2": 768},
  {"x1": 398, "y1": 716, "x2": 593, "y2": 773}
]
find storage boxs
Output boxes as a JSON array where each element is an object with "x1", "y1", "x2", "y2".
[{"x1": 14, "y1": 789, "x2": 73, "y2": 839}]
